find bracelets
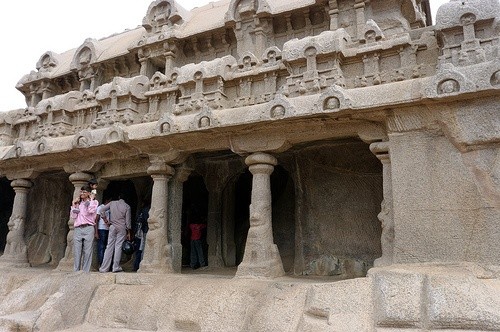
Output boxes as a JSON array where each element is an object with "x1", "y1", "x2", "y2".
[{"x1": 105, "y1": 221, "x2": 108, "y2": 223}]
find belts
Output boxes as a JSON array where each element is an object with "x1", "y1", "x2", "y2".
[{"x1": 77, "y1": 224, "x2": 91, "y2": 227}]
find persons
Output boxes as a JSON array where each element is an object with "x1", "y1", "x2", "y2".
[
  {"x1": 69, "y1": 185, "x2": 99, "y2": 271},
  {"x1": 135, "y1": 201, "x2": 151, "y2": 271},
  {"x1": 71, "y1": 178, "x2": 98, "y2": 213},
  {"x1": 99, "y1": 191, "x2": 131, "y2": 273},
  {"x1": 188, "y1": 223, "x2": 207, "y2": 270},
  {"x1": 97, "y1": 194, "x2": 111, "y2": 272}
]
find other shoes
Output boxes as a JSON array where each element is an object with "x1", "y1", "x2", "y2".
[
  {"x1": 84, "y1": 201, "x2": 90, "y2": 205},
  {"x1": 72, "y1": 209, "x2": 80, "y2": 213}
]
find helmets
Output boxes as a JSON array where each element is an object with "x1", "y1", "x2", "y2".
[{"x1": 122, "y1": 240, "x2": 137, "y2": 254}]
